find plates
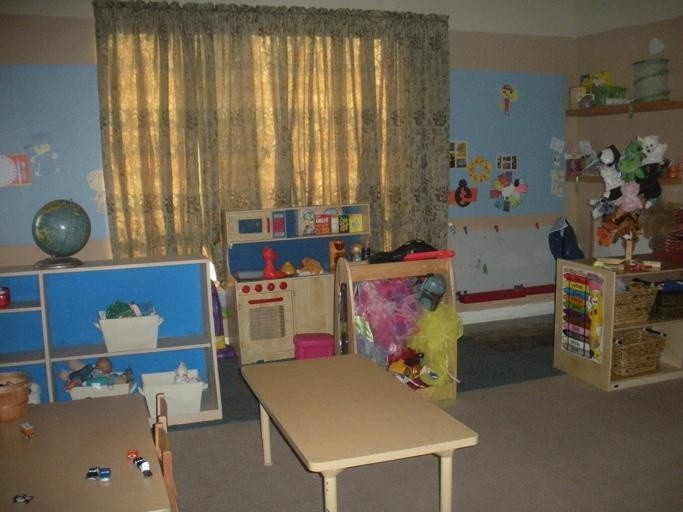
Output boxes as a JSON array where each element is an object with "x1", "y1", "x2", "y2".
[
  {"x1": 334, "y1": 251, "x2": 462, "y2": 403},
  {"x1": 0, "y1": 256, "x2": 224, "y2": 428},
  {"x1": 549, "y1": 255, "x2": 683, "y2": 396},
  {"x1": 565, "y1": 100, "x2": 683, "y2": 186},
  {"x1": 216, "y1": 202, "x2": 376, "y2": 367}
]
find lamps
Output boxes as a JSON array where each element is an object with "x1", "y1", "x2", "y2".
[{"x1": 150, "y1": 392, "x2": 182, "y2": 512}]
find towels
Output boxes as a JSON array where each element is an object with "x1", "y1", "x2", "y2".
[{"x1": 293, "y1": 334, "x2": 334, "y2": 359}]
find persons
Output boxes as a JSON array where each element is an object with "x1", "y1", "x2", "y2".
[{"x1": 54, "y1": 357, "x2": 110, "y2": 390}]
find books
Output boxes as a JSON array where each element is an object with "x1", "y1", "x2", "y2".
[{"x1": 64, "y1": 303, "x2": 207, "y2": 419}]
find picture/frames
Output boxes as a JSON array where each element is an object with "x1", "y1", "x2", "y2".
[
  {"x1": 239, "y1": 353, "x2": 481, "y2": 512},
  {"x1": 0, "y1": 390, "x2": 178, "y2": 512}
]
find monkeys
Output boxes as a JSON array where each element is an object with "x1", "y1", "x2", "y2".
[
  {"x1": 85, "y1": 466, "x2": 110, "y2": 482},
  {"x1": 19, "y1": 422, "x2": 32, "y2": 434}
]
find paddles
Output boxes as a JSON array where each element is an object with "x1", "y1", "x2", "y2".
[
  {"x1": 168, "y1": 376, "x2": 682, "y2": 512},
  {"x1": 463, "y1": 314, "x2": 553, "y2": 353}
]
